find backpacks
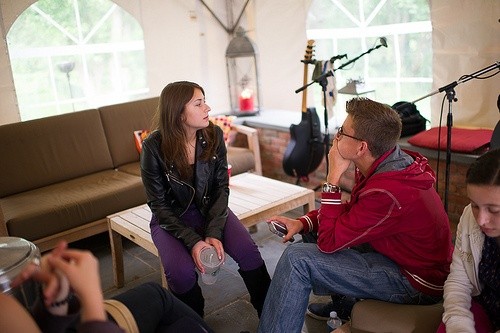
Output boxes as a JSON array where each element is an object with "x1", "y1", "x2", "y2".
[{"x1": 390, "y1": 100, "x2": 431, "y2": 139}]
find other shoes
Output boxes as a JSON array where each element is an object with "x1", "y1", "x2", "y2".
[{"x1": 306, "y1": 294, "x2": 353, "y2": 324}]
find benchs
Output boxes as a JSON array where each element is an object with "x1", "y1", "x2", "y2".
[{"x1": 0, "y1": 95, "x2": 263, "y2": 252}]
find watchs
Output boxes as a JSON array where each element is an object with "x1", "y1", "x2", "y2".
[{"x1": 322, "y1": 182, "x2": 341, "y2": 194}]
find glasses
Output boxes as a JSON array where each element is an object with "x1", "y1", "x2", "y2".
[{"x1": 337, "y1": 126, "x2": 363, "y2": 142}]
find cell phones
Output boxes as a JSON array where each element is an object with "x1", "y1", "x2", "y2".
[{"x1": 268, "y1": 221, "x2": 294, "y2": 242}]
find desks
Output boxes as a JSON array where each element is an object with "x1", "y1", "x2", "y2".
[{"x1": 107, "y1": 170, "x2": 315, "y2": 290}]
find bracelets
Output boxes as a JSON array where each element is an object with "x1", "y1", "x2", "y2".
[{"x1": 51, "y1": 295, "x2": 70, "y2": 308}]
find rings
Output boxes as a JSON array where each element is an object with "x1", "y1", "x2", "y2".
[{"x1": 222, "y1": 262, "x2": 226, "y2": 265}]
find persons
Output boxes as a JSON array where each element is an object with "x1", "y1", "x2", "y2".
[
  {"x1": 238, "y1": 97, "x2": 453, "y2": 333},
  {"x1": 0, "y1": 240, "x2": 215, "y2": 333},
  {"x1": 141, "y1": 81, "x2": 272, "y2": 325},
  {"x1": 431, "y1": 148, "x2": 500, "y2": 333}
]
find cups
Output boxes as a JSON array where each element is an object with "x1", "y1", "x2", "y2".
[
  {"x1": 198, "y1": 246, "x2": 223, "y2": 286},
  {"x1": 0, "y1": 235, "x2": 42, "y2": 311},
  {"x1": 228, "y1": 164, "x2": 232, "y2": 177}
]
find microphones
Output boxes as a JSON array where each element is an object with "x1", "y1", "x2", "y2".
[{"x1": 380, "y1": 38, "x2": 387, "y2": 47}]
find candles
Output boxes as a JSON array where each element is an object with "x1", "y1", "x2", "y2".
[{"x1": 239, "y1": 87, "x2": 255, "y2": 112}]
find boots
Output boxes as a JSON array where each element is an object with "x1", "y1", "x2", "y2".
[
  {"x1": 237, "y1": 260, "x2": 272, "y2": 319},
  {"x1": 169, "y1": 271, "x2": 206, "y2": 318}
]
